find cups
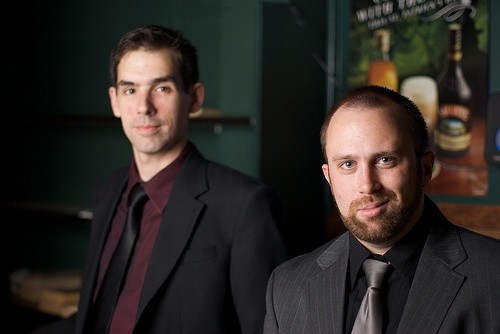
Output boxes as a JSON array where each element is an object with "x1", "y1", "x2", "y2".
[{"x1": 401, "y1": 75, "x2": 441, "y2": 182}]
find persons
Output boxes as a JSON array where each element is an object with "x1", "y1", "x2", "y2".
[
  {"x1": 261, "y1": 83, "x2": 499, "y2": 334},
  {"x1": 70, "y1": 25, "x2": 291, "y2": 334}
]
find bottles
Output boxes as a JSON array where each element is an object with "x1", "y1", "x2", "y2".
[
  {"x1": 434, "y1": 23, "x2": 472, "y2": 157},
  {"x1": 366, "y1": 29, "x2": 399, "y2": 93}
]
